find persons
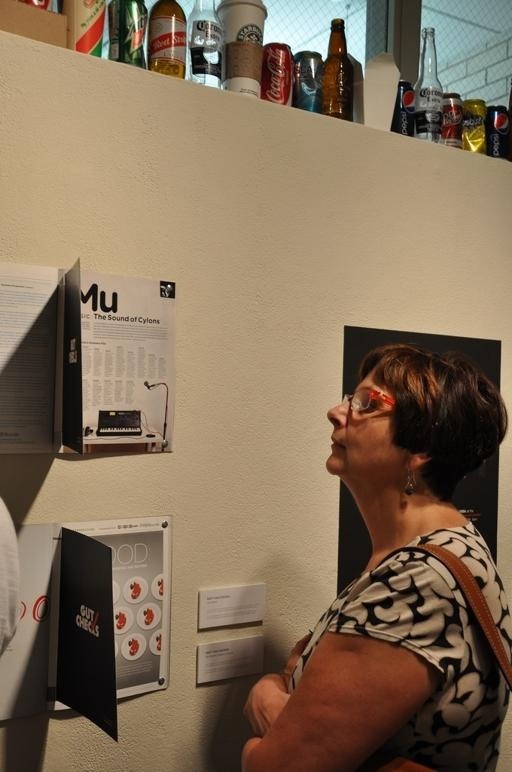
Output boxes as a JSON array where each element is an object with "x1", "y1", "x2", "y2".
[{"x1": 238, "y1": 338, "x2": 511, "y2": 769}]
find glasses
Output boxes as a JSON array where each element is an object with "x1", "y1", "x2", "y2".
[{"x1": 339, "y1": 386, "x2": 397, "y2": 413}]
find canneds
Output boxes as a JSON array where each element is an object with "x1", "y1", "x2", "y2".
[
  {"x1": 461, "y1": 99, "x2": 488, "y2": 155},
  {"x1": 106, "y1": 0, "x2": 149, "y2": 70},
  {"x1": 487, "y1": 105, "x2": 510, "y2": 159},
  {"x1": 293, "y1": 50, "x2": 324, "y2": 114},
  {"x1": 391, "y1": 79, "x2": 416, "y2": 137},
  {"x1": 263, "y1": 42, "x2": 295, "y2": 107},
  {"x1": 441, "y1": 92, "x2": 464, "y2": 150}
]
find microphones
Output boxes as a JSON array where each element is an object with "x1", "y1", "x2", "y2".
[{"x1": 144, "y1": 381, "x2": 151, "y2": 390}]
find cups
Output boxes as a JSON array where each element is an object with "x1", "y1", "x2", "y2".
[
  {"x1": 58, "y1": 1, "x2": 104, "y2": 62},
  {"x1": 215, "y1": 0, "x2": 271, "y2": 98}
]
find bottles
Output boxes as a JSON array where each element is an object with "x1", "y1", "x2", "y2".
[
  {"x1": 147, "y1": 0, "x2": 222, "y2": 89},
  {"x1": 412, "y1": 28, "x2": 444, "y2": 145},
  {"x1": 320, "y1": 17, "x2": 356, "y2": 122}
]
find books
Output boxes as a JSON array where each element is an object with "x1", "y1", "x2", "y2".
[
  {"x1": 0, "y1": 256, "x2": 180, "y2": 461},
  {"x1": 0, "y1": 519, "x2": 173, "y2": 743}
]
